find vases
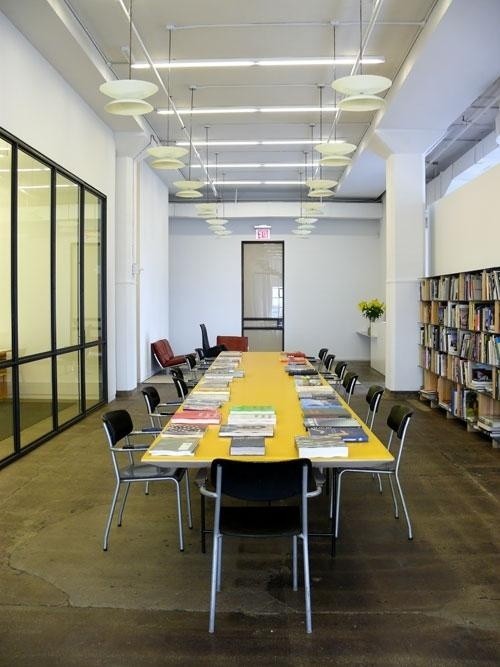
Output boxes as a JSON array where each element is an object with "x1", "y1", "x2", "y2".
[{"x1": 367, "y1": 325, "x2": 373, "y2": 335}]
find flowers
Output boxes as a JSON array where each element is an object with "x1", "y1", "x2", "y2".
[{"x1": 358, "y1": 297, "x2": 386, "y2": 323}]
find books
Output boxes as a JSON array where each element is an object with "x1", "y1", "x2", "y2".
[
  {"x1": 147, "y1": 348, "x2": 372, "y2": 464},
  {"x1": 412, "y1": 267, "x2": 499, "y2": 448}
]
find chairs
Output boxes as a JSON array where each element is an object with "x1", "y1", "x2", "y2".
[{"x1": 200, "y1": 323, "x2": 249, "y2": 368}]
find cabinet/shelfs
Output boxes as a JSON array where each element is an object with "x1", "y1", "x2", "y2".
[{"x1": 418, "y1": 266, "x2": 500, "y2": 447}]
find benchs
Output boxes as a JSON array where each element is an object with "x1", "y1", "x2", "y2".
[{"x1": 151, "y1": 338, "x2": 200, "y2": 371}]
